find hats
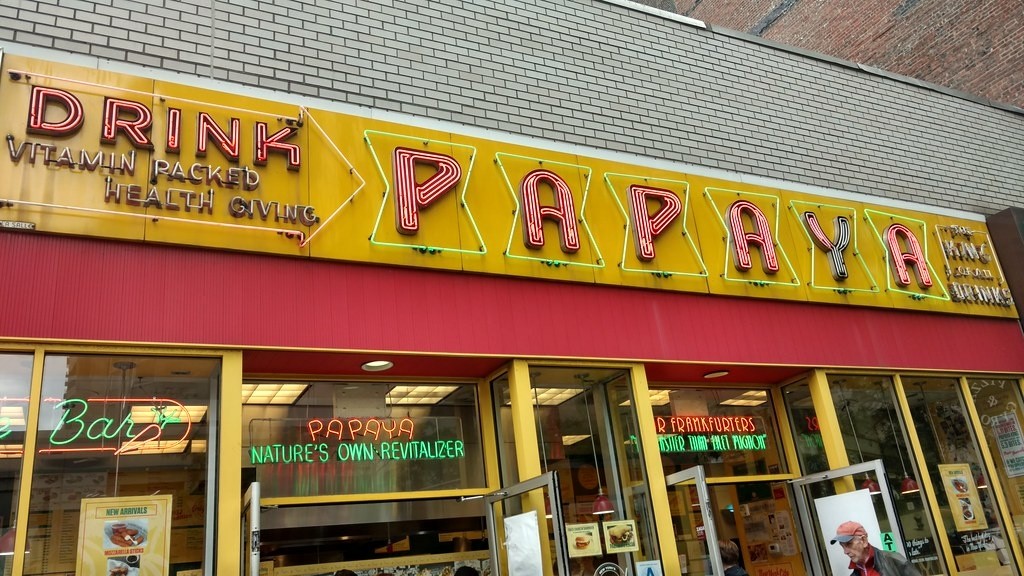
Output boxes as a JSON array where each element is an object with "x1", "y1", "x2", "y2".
[{"x1": 830, "y1": 521, "x2": 868, "y2": 546}]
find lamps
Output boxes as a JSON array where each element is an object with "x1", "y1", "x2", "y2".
[
  {"x1": 576, "y1": 372, "x2": 613, "y2": 514},
  {"x1": 978, "y1": 474, "x2": 989, "y2": 489},
  {"x1": 833, "y1": 377, "x2": 882, "y2": 496},
  {"x1": 875, "y1": 381, "x2": 920, "y2": 495}
]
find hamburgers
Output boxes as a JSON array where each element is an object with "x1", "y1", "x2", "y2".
[
  {"x1": 576, "y1": 536, "x2": 591, "y2": 549},
  {"x1": 609, "y1": 524, "x2": 633, "y2": 547}
]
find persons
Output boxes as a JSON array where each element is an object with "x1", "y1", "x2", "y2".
[
  {"x1": 454, "y1": 566, "x2": 479, "y2": 576},
  {"x1": 593, "y1": 551, "x2": 620, "y2": 576},
  {"x1": 710, "y1": 540, "x2": 750, "y2": 576},
  {"x1": 335, "y1": 571, "x2": 362, "y2": 576},
  {"x1": 830, "y1": 521, "x2": 922, "y2": 576}
]
